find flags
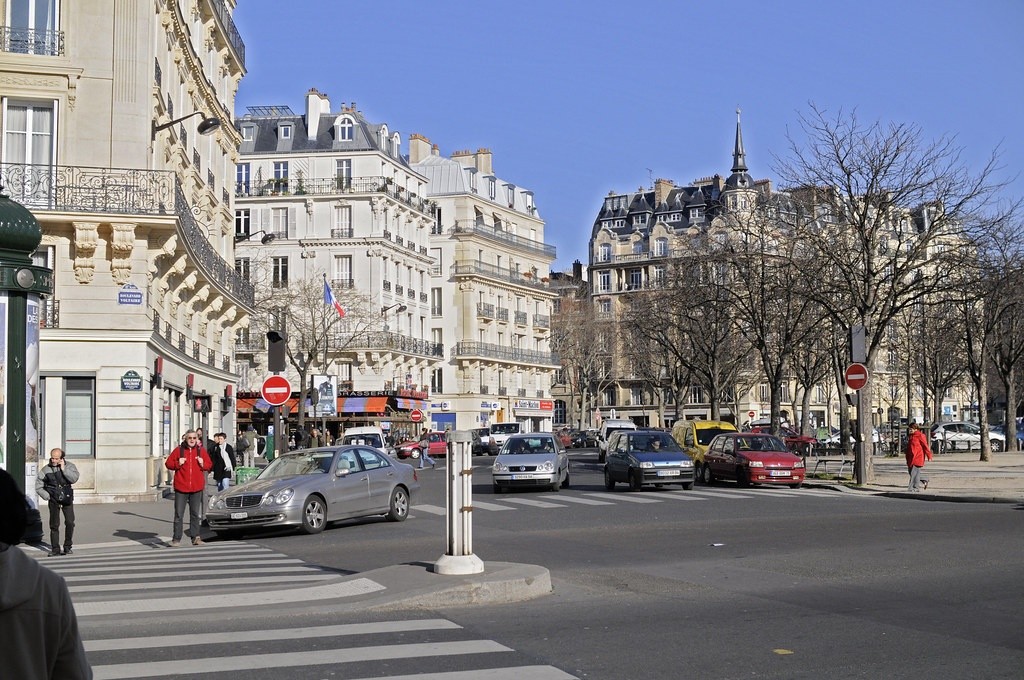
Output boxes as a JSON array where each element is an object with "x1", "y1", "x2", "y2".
[{"x1": 324, "y1": 280, "x2": 345, "y2": 318}]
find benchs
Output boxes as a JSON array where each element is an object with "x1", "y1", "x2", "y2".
[{"x1": 812, "y1": 448, "x2": 855, "y2": 480}]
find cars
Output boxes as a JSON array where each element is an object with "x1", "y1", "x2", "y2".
[
  {"x1": 927, "y1": 421, "x2": 1006, "y2": 452},
  {"x1": 205, "y1": 444, "x2": 419, "y2": 538},
  {"x1": 876, "y1": 421, "x2": 906, "y2": 439},
  {"x1": 603, "y1": 431, "x2": 694, "y2": 491},
  {"x1": 822, "y1": 426, "x2": 885, "y2": 444},
  {"x1": 701, "y1": 433, "x2": 806, "y2": 490},
  {"x1": 464, "y1": 425, "x2": 600, "y2": 456},
  {"x1": 394, "y1": 431, "x2": 447, "y2": 460},
  {"x1": 749, "y1": 427, "x2": 823, "y2": 456},
  {"x1": 991, "y1": 421, "x2": 1024, "y2": 449},
  {"x1": 491, "y1": 433, "x2": 572, "y2": 494}
]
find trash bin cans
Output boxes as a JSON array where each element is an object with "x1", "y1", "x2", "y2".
[{"x1": 236, "y1": 467, "x2": 259, "y2": 486}]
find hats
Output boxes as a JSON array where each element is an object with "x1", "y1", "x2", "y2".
[{"x1": 247, "y1": 427, "x2": 253, "y2": 431}]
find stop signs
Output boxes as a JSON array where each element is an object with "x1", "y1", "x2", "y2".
[
  {"x1": 749, "y1": 412, "x2": 755, "y2": 417},
  {"x1": 261, "y1": 376, "x2": 292, "y2": 406},
  {"x1": 410, "y1": 411, "x2": 422, "y2": 422},
  {"x1": 845, "y1": 363, "x2": 869, "y2": 390}
]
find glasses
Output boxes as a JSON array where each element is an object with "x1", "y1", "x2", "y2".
[{"x1": 188, "y1": 437, "x2": 196, "y2": 439}]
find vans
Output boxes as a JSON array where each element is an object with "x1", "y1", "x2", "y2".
[
  {"x1": 340, "y1": 427, "x2": 391, "y2": 469},
  {"x1": 596, "y1": 420, "x2": 638, "y2": 463},
  {"x1": 670, "y1": 419, "x2": 751, "y2": 487}
]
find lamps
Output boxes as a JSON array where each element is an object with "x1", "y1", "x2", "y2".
[
  {"x1": 381, "y1": 303, "x2": 407, "y2": 316},
  {"x1": 152, "y1": 112, "x2": 220, "y2": 141},
  {"x1": 233, "y1": 230, "x2": 275, "y2": 249}
]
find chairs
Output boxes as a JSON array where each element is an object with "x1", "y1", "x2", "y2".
[{"x1": 337, "y1": 459, "x2": 351, "y2": 469}]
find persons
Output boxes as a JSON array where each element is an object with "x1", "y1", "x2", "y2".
[
  {"x1": 165, "y1": 430, "x2": 212, "y2": 547},
  {"x1": 514, "y1": 438, "x2": 554, "y2": 454},
  {"x1": 417, "y1": 428, "x2": 436, "y2": 468},
  {"x1": 905, "y1": 422, "x2": 933, "y2": 492},
  {"x1": 294, "y1": 425, "x2": 335, "y2": 450},
  {"x1": 35, "y1": 448, "x2": 80, "y2": 557},
  {"x1": 182, "y1": 425, "x2": 261, "y2": 492},
  {"x1": 646, "y1": 435, "x2": 666, "y2": 450},
  {"x1": 0, "y1": 468, "x2": 97, "y2": 680}
]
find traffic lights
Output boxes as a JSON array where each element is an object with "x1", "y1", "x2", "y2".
[{"x1": 266, "y1": 331, "x2": 286, "y2": 372}]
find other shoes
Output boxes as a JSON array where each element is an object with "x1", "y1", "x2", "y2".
[
  {"x1": 165, "y1": 539, "x2": 181, "y2": 547},
  {"x1": 923, "y1": 479, "x2": 929, "y2": 489},
  {"x1": 192, "y1": 538, "x2": 206, "y2": 545},
  {"x1": 48, "y1": 551, "x2": 61, "y2": 557},
  {"x1": 432, "y1": 463, "x2": 436, "y2": 468},
  {"x1": 64, "y1": 549, "x2": 73, "y2": 555},
  {"x1": 417, "y1": 467, "x2": 423, "y2": 469}
]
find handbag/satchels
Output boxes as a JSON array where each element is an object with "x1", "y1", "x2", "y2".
[
  {"x1": 418, "y1": 439, "x2": 428, "y2": 447},
  {"x1": 237, "y1": 437, "x2": 250, "y2": 452}
]
